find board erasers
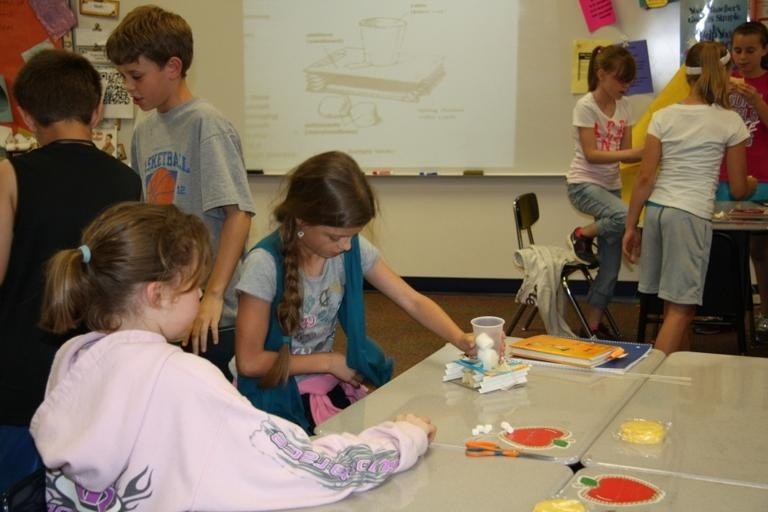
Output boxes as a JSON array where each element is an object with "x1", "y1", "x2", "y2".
[
  {"x1": 246, "y1": 169, "x2": 264, "y2": 174},
  {"x1": 463, "y1": 170, "x2": 484, "y2": 175}
]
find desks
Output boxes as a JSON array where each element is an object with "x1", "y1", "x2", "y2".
[
  {"x1": 274, "y1": 436, "x2": 574, "y2": 510},
  {"x1": 579, "y1": 351, "x2": 764, "y2": 467},
  {"x1": 632, "y1": 200, "x2": 767, "y2": 355},
  {"x1": 547, "y1": 467, "x2": 764, "y2": 512},
  {"x1": 313, "y1": 332, "x2": 665, "y2": 468}
]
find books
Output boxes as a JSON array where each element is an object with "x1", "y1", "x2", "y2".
[{"x1": 509, "y1": 335, "x2": 652, "y2": 374}]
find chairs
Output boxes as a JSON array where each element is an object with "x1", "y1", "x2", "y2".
[
  {"x1": 0, "y1": 465, "x2": 48, "y2": 512},
  {"x1": 178, "y1": 325, "x2": 236, "y2": 385},
  {"x1": 505, "y1": 192, "x2": 624, "y2": 341}
]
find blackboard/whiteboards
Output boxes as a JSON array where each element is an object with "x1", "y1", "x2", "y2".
[{"x1": 117, "y1": 0, "x2": 681, "y2": 178}]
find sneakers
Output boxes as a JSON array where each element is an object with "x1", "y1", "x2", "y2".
[
  {"x1": 580, "y1": 322, "x2": 616, "y2": 341},
  {"x1": 566, "y1": 227, "x2": 596, "y2": 265}
]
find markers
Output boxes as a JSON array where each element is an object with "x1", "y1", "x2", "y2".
[
  {"x1": 371, "y1": 169, "x2": 392, "y2": 176},
  {"x1": 419, "y1": 172, "x2": 438, "y2": 176}
]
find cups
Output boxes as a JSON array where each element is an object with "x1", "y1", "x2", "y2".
[{"x1": 469, "y1": 316, "x2": 506, "y2": 361}]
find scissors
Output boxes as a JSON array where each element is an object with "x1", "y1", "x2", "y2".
[{"x1": 464, "y1": 440, "x2": 559, "y2": 461}]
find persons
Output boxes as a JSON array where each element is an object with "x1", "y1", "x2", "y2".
[
  {"x1": 0, "y1": 47, "x2": 145, "y2": 512},
  {"x1": 566, "y1": 46, "x2": 646, "y2": 341},
  {"x1": 106, "y1": 5, "x2": 256, "y2": 384},
  {"x1": 234, "y1": 151, "x2": 506, "y2": 438},
  {"x1": 693, "y1": 22, "x2": 768, "y2": 348},
  {"x1": 27, "y1": 203, "x2": 436, "y2": 512},
  {"x1": 622, "y1": 41, "x2": 758, "y2": 357}
]
